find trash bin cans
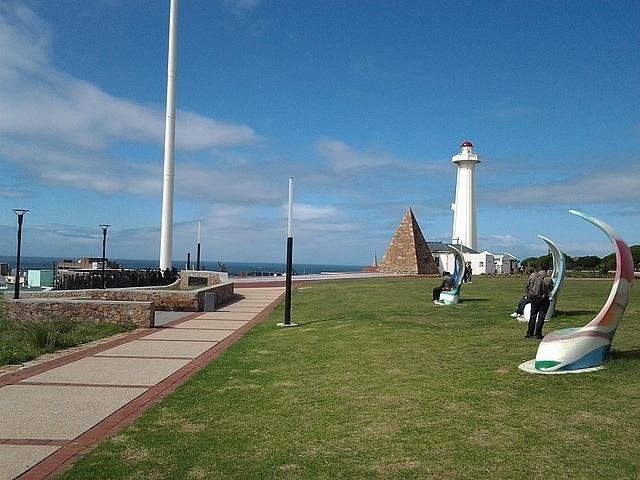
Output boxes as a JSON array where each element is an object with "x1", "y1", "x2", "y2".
[{"x1": 204, "y1": 293, "x2": 216, "y2": 312}]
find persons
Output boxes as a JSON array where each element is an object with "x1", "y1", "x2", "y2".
[
  {"x1": 523, "y1": 261, "x2": 555, "y2": 339},
  {"x1": 431, "y1": 257, "x2": 473, "y2": 306},
  {"x1": 61, "y1": 267, "x2": 178, "y2": 290},
  {"x1": 509, "y1": 266, "x2": 537, "y2": 321}
]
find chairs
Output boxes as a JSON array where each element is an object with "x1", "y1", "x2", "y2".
[
  {"x1": 519, "y1": 235, "x2": 566, "y2": 323},
  {"x1": 434, "y1": 244, "x2": 466, "y2": 306},
  {"x1": 533, "y1": 209, "x2": 635, "y2": 372}
]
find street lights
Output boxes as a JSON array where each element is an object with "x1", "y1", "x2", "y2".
[
  {"x1": 100, "y1": 224, "x2": 109, "y2": 288},
  {"x1": 11, "y1": 207, "x2": 30, "y2": 300}
]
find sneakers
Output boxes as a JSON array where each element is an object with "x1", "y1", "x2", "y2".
[{"x1": 510, "y1": 312, "x2": 521, "y2": 317}]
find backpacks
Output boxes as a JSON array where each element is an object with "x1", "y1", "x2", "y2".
[{"x1": 529, "y1": 275, "x2": 547, "y2": 303}]
